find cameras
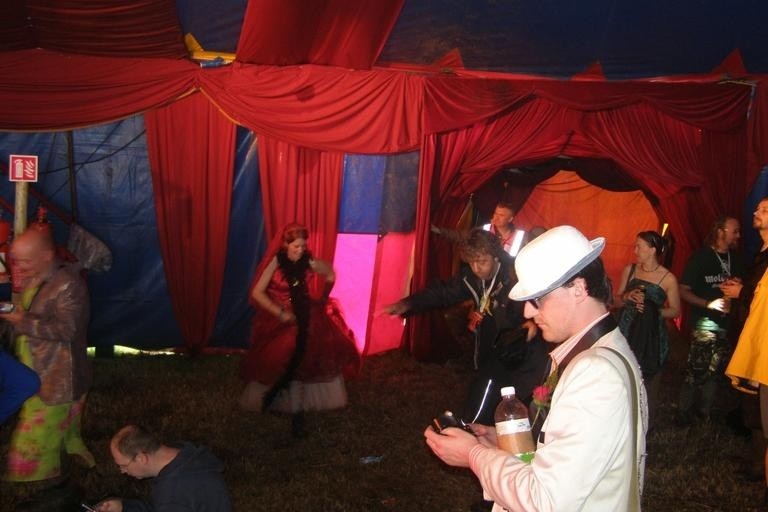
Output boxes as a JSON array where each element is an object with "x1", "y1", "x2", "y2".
[
  {"x1": 0, "y1": 302, "x2": 17, "y2": 314},
  {"x1": 432, "y1": 410, "x2": 466, "y2": 438}
]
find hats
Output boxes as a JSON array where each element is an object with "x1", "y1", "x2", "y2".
[{"x1": 509, "y1": 225, "x2": 607, "y2": 302}]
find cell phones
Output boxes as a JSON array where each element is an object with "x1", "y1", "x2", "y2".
[{"x1": 81, "y1": 502, "x2": 94, "y2": 511}]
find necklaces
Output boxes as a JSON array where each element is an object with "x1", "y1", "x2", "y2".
[{"x1": 641, "y1": 264, "x2": 660, "y2": 272}]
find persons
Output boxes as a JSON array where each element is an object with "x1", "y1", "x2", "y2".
[
  {"x1": 609, "y1": 229, "x2": 683, "y2": 399},
  {"x1": 429, "y1": 200, "x2": 529, "y2": 274},
  {"x1": 239, "y1": 220, "x2": 350, "y2": 435},
  {"x1": 724, "y1": 263, "x2": 768, "y2": 488},
  {"x1": 423, "y1": 223, "x2": 650, "y2": 511},
  {"x1": 668, "y1": 212, "x2": 745, "y2": 427},
  {"x1": 718, "y1": 196, "x2": 768, "y2": 440},
  {"x1": 3, "y1": 229, "x2": 97, "y2": 498},
  {"x1": 524, "y1": 225, "x2": 548, "y2": 246},
  {"x1": 85, "y1": 422, "x2": 237, "y2": 512},
  {"x1": 370, "y1": 227, "x2": 551, "y2": 418}
]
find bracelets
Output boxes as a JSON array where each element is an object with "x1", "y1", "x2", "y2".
[
  {"x1": 704, "y1": 298, "x2": 711, "y2": 311},
  {"x1": 620, "y1": 291, "x2": 629, "y2": 306},
  {"x1": 278, "y1": 306, "x2": 285, "y2": 319},
  {"x1": 321, "y1": 289, "x2": 329, "y2": 302}
]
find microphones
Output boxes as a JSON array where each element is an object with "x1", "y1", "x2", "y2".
[{"x1": 639, "y1": 283, "x2": 647, "y2": 307}]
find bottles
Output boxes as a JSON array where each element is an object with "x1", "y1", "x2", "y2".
[
  {"x1": 634, "y1": 284, "x2": 646, "y2": 310},
  {"x1": 493, "y1": 384, "x2": 535, "y2": 466}
]
